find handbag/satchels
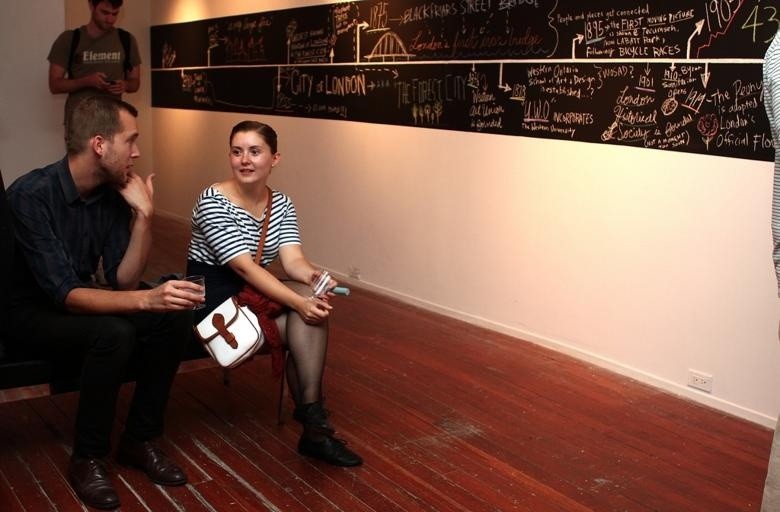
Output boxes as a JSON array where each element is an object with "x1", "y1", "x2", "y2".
[{"x1": 192, "y1": 295, "x2": 265, "y2": 371}]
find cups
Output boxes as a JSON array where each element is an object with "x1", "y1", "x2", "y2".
[
  {"x1": 180, "y1": 275, "x2": 212, "y2": 312},
  {"x1": 307, "y1": 268, "x2": 334, "y2": 298}
]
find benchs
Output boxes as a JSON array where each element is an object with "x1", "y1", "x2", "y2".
[{"x1": 0, "y1": 344, "x2": 287, "y2": 426}]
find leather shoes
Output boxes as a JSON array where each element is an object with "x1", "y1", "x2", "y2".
[
  {"x1": 113, "y1": 436, "x2": 187, "y2": 487},
  {"x1": 298, "y1": 426, "x2": 363, "y2": 466},
  {"x1": 293, "y1": 403, "x2": 338, "y2": 434},
  {"x1": 66, "y1": 456, "x2": 121, "y2": 509}
]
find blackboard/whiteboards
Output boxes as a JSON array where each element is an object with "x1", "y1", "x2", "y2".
[{"x1": 150, "y1": 0, "x2": 780, "y2": 162}]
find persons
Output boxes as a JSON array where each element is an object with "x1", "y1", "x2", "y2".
[
  {"x1": 47, "y1": 0, "x2": 142, "y2": 126},
  {"x1": 1, "y1": 85, "x2": 208, "y2": 511},
  {"x1": 185, "y1": 120, "x2": 364, "y2": 467}
]
